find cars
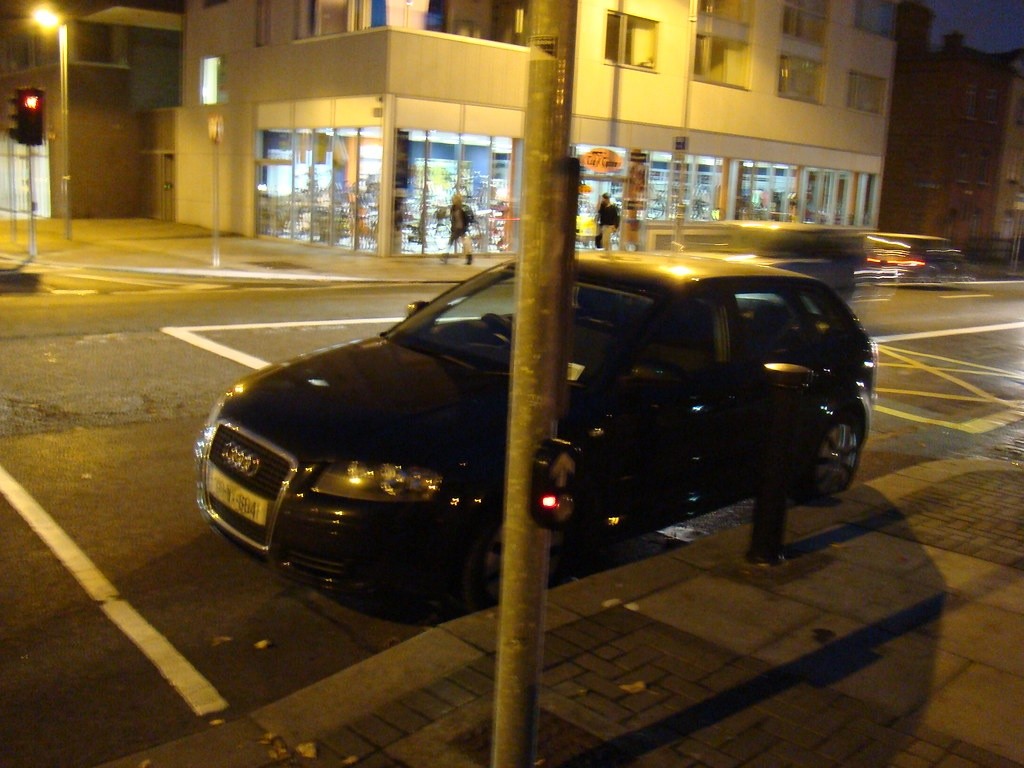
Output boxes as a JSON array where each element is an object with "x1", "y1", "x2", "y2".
[
  {"x1": 192, "y1": 249, "x2": 878, "y2": 622},
  {"x1": 729, "y1": 229, "x2": 864, "y2": 293}
]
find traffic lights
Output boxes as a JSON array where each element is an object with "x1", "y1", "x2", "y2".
[{"x1": 7, "y1": 87, "x2": 45, "y2": 146}]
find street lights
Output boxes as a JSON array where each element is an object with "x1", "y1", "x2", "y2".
[{"x1": 33, "y1": 7, "x2": 72, "y2": 238}]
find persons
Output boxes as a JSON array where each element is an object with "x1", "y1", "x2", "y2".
[
  {"x1": 439, "y1": 195, "x2": 476, "y2": 265},
  {"x1": 596, "y1": 191, "x2": 622, "y2": 252}
]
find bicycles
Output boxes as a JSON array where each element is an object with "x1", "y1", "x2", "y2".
[{"x1": 257, "y1": 165, "x2": 817, "y2": 253}]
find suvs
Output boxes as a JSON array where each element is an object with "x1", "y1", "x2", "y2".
[{"x1": 860, "y1": 232, "x2": 966, "y2": 282}]
